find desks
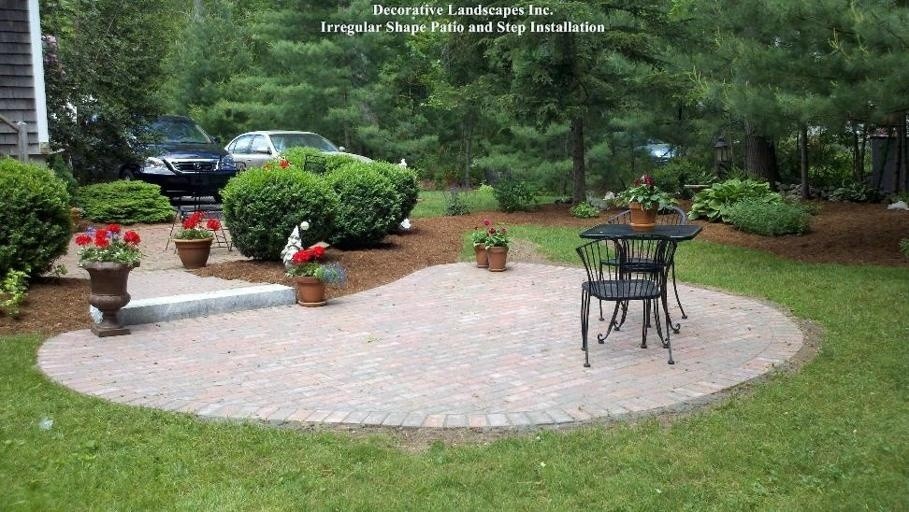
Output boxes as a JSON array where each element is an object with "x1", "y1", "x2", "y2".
[{"x1": 580, "y1": 223, "x2": 703, "y2": 348}]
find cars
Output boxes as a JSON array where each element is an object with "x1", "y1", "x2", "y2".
[
  {"x1": 632, "y1": 143, "x2": 685, "y2": 166},
  {"x1": 221, "y1": 129, "x2": 379, "y2": 184},
  {"x1": 795, "y1": 120, "x2": 872, "y2": 143}
]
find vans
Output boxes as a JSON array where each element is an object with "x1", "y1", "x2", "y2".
[{"x1": 87, "y1": 109, "x2": 236, "y2": 204}]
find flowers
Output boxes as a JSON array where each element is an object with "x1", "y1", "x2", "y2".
[
  {"x1": 481, "y1": 226, "x2": 511, "y2": 246},
  {"x1": 603, "y1": 173, "x2": 674, "y2": 209},
  {"x1": 74, "y1": 221, "x2": 147, "y2": 263},
  {"x1": 473, "y1": 219, "x2": 491, "y2": 245},
  {"x1": 172, "y1": 208, "x2": 224, "y2": 240},
  {"x1": 289, "y1": 245, "x2": 350, "y2": 286}
]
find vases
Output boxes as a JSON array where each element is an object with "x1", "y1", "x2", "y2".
[
  {"x1": 294, "y1": 276, "x2": 328, "y2": 305},
  {"x1": 473, "y1": 243, "x2": 490, "y2": 269},
  {"x1": 485, "y1": 247, "x2": 509, "y2": 272},
  {"x1": 174, "y1": 238, "x2": 214, "y2": 269},
  {"x1": 80, "y1": 259, "x2": 141, "y2": 337},
  {"x1": 629, "y1": 200, "x2": 657, "y2": 229}
]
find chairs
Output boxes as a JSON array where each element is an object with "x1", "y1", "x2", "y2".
[
  {"x1": 577, "y1": 231, "x2": 677, "y2": 369},
  {"x1": 597, "y1": 203, "x2": 691, "y2": 322}
]
